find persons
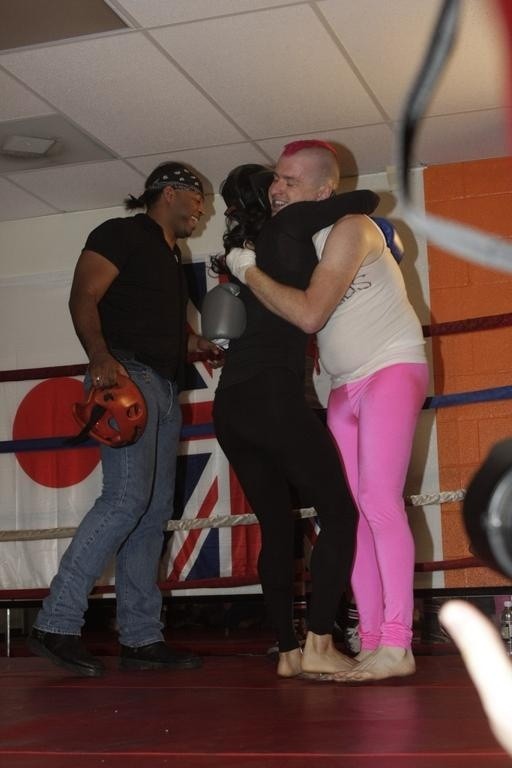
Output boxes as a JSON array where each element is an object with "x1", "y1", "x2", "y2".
[
  {"x1": 28, "y1": 161, "x2": 226, "y2": 674},
  {"x1": 222, "y1": 141, "x2": 428, "y2": 684},
  {"x1": 213, "y1": 163, "x2": 385, "y2": 678}
]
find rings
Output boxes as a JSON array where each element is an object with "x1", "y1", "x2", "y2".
[{"x1": 95, "y1": 377, "x2": 101, "y2": 382}]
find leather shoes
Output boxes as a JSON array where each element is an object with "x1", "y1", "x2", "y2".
[
  {"x1": 119, "y1": 643, "x2": 203, "y2": 671},
  {"x1": 26, "y1": 630, "x2": 107, "y2": 678}
]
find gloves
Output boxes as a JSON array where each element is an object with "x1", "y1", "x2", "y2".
[{"x1": 224, "y1": 246, "x2": 257, "y2": 284}]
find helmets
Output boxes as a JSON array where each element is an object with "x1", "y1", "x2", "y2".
[{"x1": 72, "y1": 381, "x2": 148, "y2": 447}]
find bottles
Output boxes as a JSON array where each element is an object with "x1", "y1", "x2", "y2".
[{"x1": 501, "y1": 601, "x2": 512, "y2": 655}]
